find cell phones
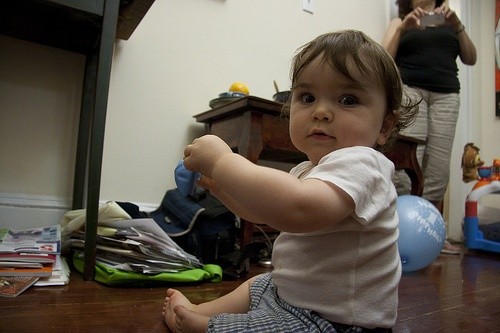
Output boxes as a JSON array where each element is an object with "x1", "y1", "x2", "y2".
[{"x1": 420, "y1": 12, "x2": 445, "y2": 26}]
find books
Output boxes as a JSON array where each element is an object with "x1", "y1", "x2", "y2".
[
  {"x1": 0, "y1": 224, "x2": 71, "y2": 297},
  {"x1": 61, "y1": 200, "x2": 205, "y2": 275}
]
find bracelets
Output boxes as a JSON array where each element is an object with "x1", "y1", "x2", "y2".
[{"x1": 453, "y1": 24, "x2": 465, "y2": 35}]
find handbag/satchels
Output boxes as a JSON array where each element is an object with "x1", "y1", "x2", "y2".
[{"x1": 150, "y1": 186, "x2": 250, "y2": 280}]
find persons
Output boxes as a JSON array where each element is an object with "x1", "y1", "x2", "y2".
[
  {"x1": 161, "y1": 30, "x2": 402, "y2": 333},
  {"x1": 382, "y1": 0, "x2": 477, "y2": 254}
]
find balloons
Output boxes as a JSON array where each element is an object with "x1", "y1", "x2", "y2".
[{"x1": 397, "y1": 195, "x2": 445, "y2": 272}]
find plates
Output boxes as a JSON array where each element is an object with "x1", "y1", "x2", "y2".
[{"x1": 209, "y1": 96, "x2": 242, "y2": 109}]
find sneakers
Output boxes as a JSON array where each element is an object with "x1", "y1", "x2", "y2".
[{"x1": 440, "y1": 240, "x2": 462, "y2": 253}]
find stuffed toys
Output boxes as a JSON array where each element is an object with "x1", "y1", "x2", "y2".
[{"x1": 461, "y1": 145, "x2": 484, "y2": 182}]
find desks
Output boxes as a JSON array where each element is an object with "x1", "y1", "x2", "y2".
[
  {"x1": 0, "y1": 0, "x2": 155, "y2": 282},
  {"x1": 194, "y1": 96, "x2": 427, "y2": 279}
]
